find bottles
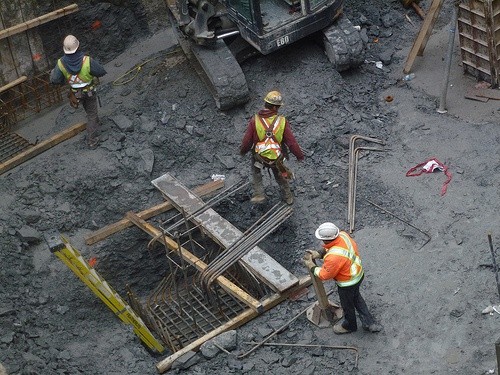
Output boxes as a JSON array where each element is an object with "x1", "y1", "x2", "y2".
[{"x1": 402, "y1": 72, "x2": 416, "y2": 81}]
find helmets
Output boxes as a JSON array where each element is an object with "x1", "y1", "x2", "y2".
[
  {"x1": 314, "y1": 222, "x2": 339, "y2": 240},
  {"x1": 263, "y1": 90, "x2": 284, "y2": 106},
  {"x1": 62, "y1": 34, "x2": 80, "y2": 54}
]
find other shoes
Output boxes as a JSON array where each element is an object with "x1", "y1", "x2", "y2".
[
  {"x1": 282, "y1": 197, "x2": 294, "y2": 205},
  {"x1": 250, "y1": 195, "x2": 267, "y2": 204},
  {"x1": 88, "y1": 136, "x2": 100, "y2": 150},
  {"x1": 332, "y1": 323, "x2": 355, "y2": 335}
]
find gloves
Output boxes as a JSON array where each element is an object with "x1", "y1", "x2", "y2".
[
  {"x1": 303, "y1": 250, "x2": 321, "y2": 260},
  {"x1": 300, "y1": 254, "x2": 317, "y2": 271}
]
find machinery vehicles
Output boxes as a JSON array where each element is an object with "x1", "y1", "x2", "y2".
[{"x1": 162, "y1": 0, "x2": 366, "y2": 111}]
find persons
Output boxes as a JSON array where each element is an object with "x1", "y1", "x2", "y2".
[
  {"x1": 50, "y1": 35, "x2": 108, "y2": 149},
  {"x1": 302, "y1": 222, "x2": 382, "y2": 334},
  {"x1": 239, "y1": 91, "x2": 305, "y2": 204}
]
